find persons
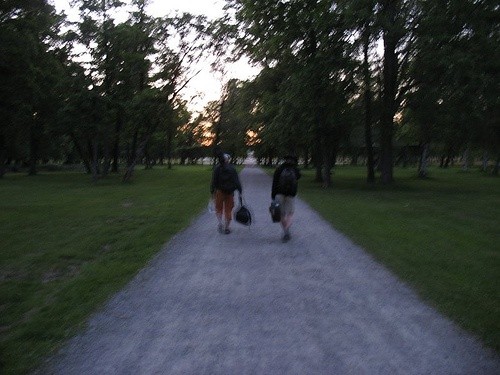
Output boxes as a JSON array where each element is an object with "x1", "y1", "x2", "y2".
[
  {"x1": 210, "y1": 152, "x2": 243, "y2": 234},
  {"x1": 271, "y1": 154, "x2": 303, "y2": 243}
]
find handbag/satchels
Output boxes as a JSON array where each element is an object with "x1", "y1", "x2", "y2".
[
  {"x1": 234, "y1": 198, "x2": 251, "y2": 226},
  {"x1": 270, "y1": 201, "x2": 281, "y2": 223}
]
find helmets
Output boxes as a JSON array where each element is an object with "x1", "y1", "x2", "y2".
[{"x1": 219, "y1": 154, "x2": 230, "y2": 164}]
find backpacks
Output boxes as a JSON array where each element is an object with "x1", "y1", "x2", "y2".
[
  {"x1": 278, "y1": 167, "x2": 297, "y2": 197},
  {"x1": 218, "y1": 166, "x2": 236, "y2": 195}
]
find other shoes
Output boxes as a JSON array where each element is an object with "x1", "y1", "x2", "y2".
[
  {"x1": 216, "y1": 224, "x2": 231, "y2": 235},
  {"x1": 280, "y1": 232, "x2": 291, "y2": 242}
]
